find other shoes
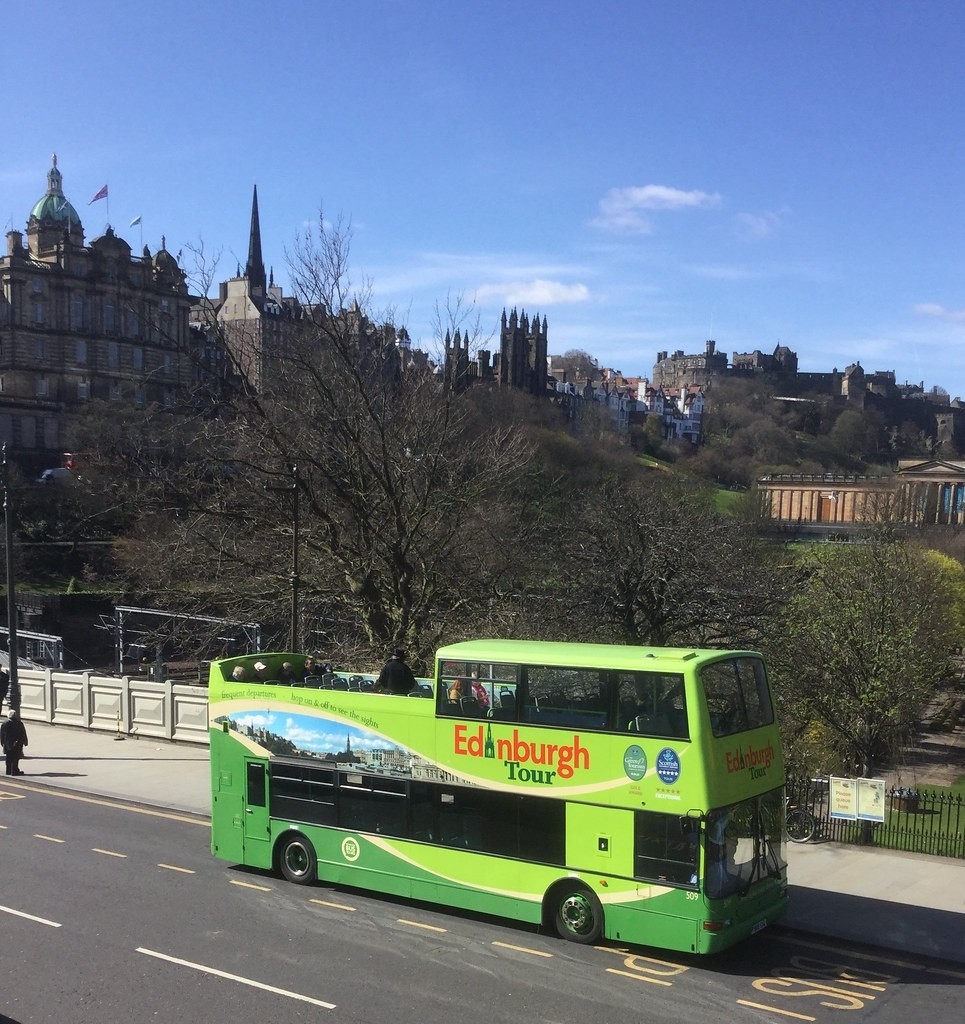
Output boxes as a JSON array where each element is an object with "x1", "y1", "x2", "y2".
[
  {"x1": 5, "y1": 772, "x2": 10, "y2": 775},
  {"x1": 10, "y1": 771, "x2": 23, "y2": 776}
]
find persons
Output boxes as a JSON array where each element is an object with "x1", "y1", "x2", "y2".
[
  {"x1": 0, "y1": 664, "x2": 9, "y2": 714},
  {"x1": 378, "y1": 649, "x2": 415, "y2": 695},
  {"x1": 0, "y1": 709, "x2": 28, "y2": 776},
  {"x1": 227, "y1": 658, "x2": 333, "y2": 684},
  {"x1": 448, "y1": 671, "x2": 490, "y2": 706}
]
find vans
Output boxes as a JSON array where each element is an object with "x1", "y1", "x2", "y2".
[{"x1": 34, "y1": 468, "x2": 79, "y2": 487}]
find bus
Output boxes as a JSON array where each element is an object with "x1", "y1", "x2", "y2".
[
  {"x1": 59, "y1": 452, "x2": 127, "y2": 470},
  {"x1": 202, "y1": 638, "x2": 791, "y2": 958}
]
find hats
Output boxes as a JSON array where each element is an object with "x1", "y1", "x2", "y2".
[
  {"x1": 252, "y1": 661, "x2": 265, "y2": 671},
  {"x1": 392, "y1": 648, "x2": 404, "y2": 657}
]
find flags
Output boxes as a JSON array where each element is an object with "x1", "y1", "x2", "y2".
[
  {"x1": 56, "y1": 201, "x2": 68, "y2": 215},
  {"x1": 130, "y1": 217, "x2": 140, "y2": 227},
  {"x1": 177, "y1": 250, "x2": 182, "y2": 262},
  {"x1": 88, "y1": 185, "x2": 107, "y2": 205}
]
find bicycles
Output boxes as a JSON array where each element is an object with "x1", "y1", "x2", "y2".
[{"x1": 733, "y1": 790, "x2": 816, "y2": 843}]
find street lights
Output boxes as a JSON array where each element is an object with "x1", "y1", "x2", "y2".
[{"x1": 264, "y1": 483, "x2": 297, "y2": 652}]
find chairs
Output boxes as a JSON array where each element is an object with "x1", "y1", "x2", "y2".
[{"x1": 263, "y1": 673, "x2": 691, "y2": 740}]
